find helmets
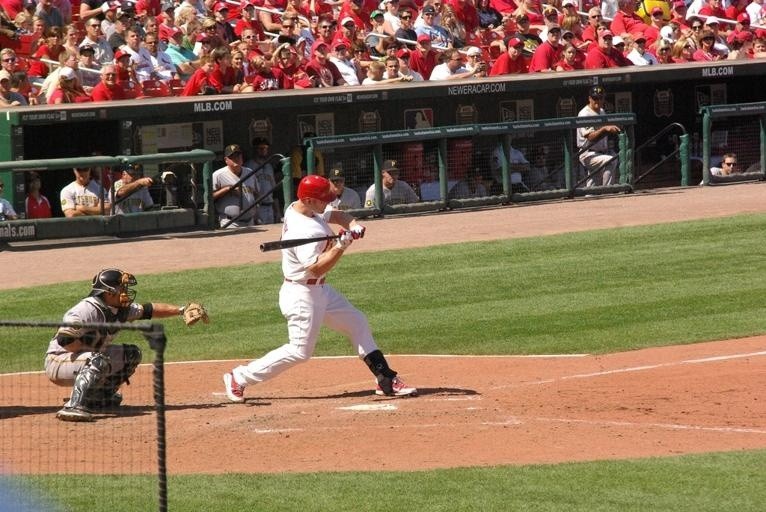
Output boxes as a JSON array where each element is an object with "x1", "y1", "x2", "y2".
[
  {"x1": 90, "y1": 268, "x2": 137, "y2": 308},
  {"x1": 297, "y1": 175, "x2": 337, "y2": 202}
]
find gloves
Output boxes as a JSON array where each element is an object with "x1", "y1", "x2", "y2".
[{"x1": 335, "y1": 224, "x2": 366, "y2": 252}]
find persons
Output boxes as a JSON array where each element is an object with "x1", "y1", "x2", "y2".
[
  {"x1": 223, "y1": 175, "x2": 416, "y2": 404},
  {"x1": 44, "y1": 269, "x2": 203, "y2": 422}
]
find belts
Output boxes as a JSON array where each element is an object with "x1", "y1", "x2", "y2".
[{"x1": 286, "y1": 277, "x2": 325, "y2": 284}]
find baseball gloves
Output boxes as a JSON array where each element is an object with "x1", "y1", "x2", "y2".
[{"x1": 183, "y1": 302, "x2": 208, "y2": 328}]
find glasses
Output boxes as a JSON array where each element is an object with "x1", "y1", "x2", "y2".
[{"x1": 725, "y1": 162, "x2": 737, "y2": 167}]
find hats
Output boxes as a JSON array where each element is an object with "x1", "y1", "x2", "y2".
[
  {"x1": 0, "y1": 69, "x2": 12, "y2": 82},
  {"x1": 252, "y1": 137, "x2": 270, "y2": 146},
  {"x1": 328, "y1": 168, "x2": 345, "y2": 181},
  {"x1": 58, "y1": 66, "x2": 76, "y2": 80},
  {"x1": 467, "y1": 46, "x2": 484, "y2": 57},
  {"x1": 507, "y1": 0, "x2": 720, "y2": 46},
  {"x1": 224, "y1": 144, "x2": 242, "y2": 157},
  {"x1": 589, "y1": 86, "x2": 607, "y2": 98},
  {"x1": 101, "y1": 0, "x2": 136, "y2": 23},
  {"x1": 196, "y1": 0, "x2": 435, "y2": 57},
  {"x1": 167, "y1": 26, "x2": 183, "y2": 38},
  {"x1": 79, "y1": 46, "x2": 95, "y2": 56},
  {"x1": 382, "y1": 160, "x2": 400, "y2": 172},
  {"x1": 112, "y1": 50, "x2": 132, "y2": 63},
  {"x1": 161, "y1": 2, "x2": 175, "y2": 12}
]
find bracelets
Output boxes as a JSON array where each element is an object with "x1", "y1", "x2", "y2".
[{"x1": 178, "y1": 305, "x2": 185, "y2": 316}]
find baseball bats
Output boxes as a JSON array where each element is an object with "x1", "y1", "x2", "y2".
[{"x1": 259, "y1": 236, "x2": 341, "y2": 252}]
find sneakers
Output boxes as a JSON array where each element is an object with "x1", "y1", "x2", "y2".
[
  {"x1": 223, "y1": 373, "x2": 245, "y2": 403},
  {"x1": 375, "y1": 376, "x2": 418, "y2": 395},
  {"x1": 56, "y1": 407, "x2": 93, "y2": 422},
  {"x1": 92, "y1": 392, "x2": 122, "y2": 406}
]
